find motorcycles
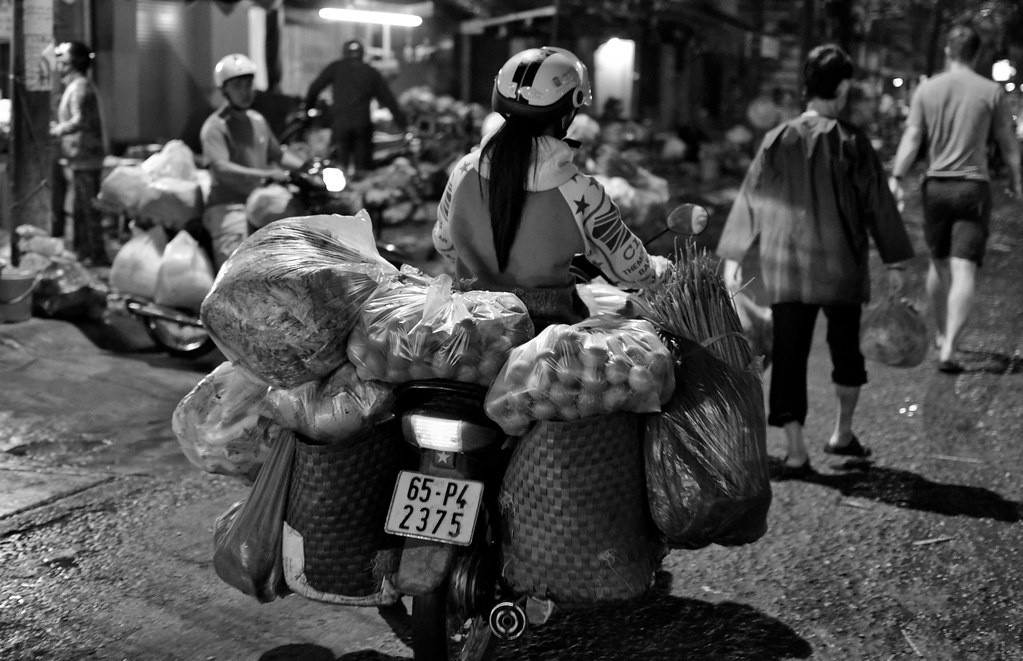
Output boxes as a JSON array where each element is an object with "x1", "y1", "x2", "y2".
[
  {"x1": 380, "y1": 198, "x2": 711, "y2": 661},
  {"x1": 122, "y1": 150, "x2": 416, "y2": 366}
]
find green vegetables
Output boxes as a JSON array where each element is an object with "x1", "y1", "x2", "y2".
[{"x1": 200, "y1": 221, "x2": 380, "y2": 388}]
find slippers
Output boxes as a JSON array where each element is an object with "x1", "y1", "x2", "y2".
[
  {"x1": 781, "y1": 453, "x2": 811, "y2": 478},
  {"x1": 935, "y1": 330, "x2": 945, "y2": 351},
  {"x1": 940, "y1": 360, "x2": 962, "y2": 373},
  {"x1": 824, "y1": 435, "x2": 872, "y2": 458}
]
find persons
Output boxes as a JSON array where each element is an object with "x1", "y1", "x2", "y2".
[
  {"x1": 717, "y1": 44, "x2": 918, "y2": 477},
  {"x1": 199, "y1": 53, "x2": 323, "y2": 271},
  {"x1": 432, "y1": 47, "x2": 677, "y2": 333},
  {"x1": 36, "y1": 39, "x2": 112, "y2": 268},
  {"x1": 887, "y1": 25, "x2": 1023, "y2": 373},
  {"x1": 306, "y1": 40, "x2": 414, "y2": 175},
  {"x1": 591, "y1": 97, "x2": 652, "y2": 160}
]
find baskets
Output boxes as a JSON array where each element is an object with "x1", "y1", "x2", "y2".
[
  {"x1": 500, "y1": 408, "x2": 660, "y2": 603},
  {"x1": 282, "y1": 413, "x2": 400, "y2": 604}
]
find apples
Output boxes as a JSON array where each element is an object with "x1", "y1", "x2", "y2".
[
  {"x1": 344, "y1": 294, "x2": 532, "y2": 386},
  {"x1": 487, "y1": 321, "x2": 674, "y2": 436}
]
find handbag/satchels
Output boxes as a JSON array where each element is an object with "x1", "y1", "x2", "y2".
[
  {"x1": 108, "y1": 223, "x2": 167, "y2": 300},
  {"x1": 641, "y1": 320, "x2": 771, "y2": 547},
  {"x1": 156, "y1": 231, "x2": 213, "y2": 310},
  {"x1": 859, "y1": 290, "x2": 929, "y2": 367},
  {"x1": 213, "y1": 429, "x2": 294, "y2": 605},
  {"x1": 724, "y1": 287, "x2": 773, "y2": 375}
]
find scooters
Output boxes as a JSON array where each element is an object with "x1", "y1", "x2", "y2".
[{"x1": 277, "y1": 107, "x2": 419, "y2": 225}]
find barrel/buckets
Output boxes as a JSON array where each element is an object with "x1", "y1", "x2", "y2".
[{"x1": 0, "y1": 267, "x2": 43, "y2": 324}]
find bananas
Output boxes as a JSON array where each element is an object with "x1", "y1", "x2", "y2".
[{"x1": 172, "y1": 369, "x2": 280, "y2": 474}]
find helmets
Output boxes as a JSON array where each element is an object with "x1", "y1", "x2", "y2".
[
  {"x1": 57, "y1": 42, "x2": 90, "y2": 72},
  {"x1": 213, "y1": 53, "x2": 254, "y2": 88},
  {"x1": 344, "y1": 41, "x2": 362, "y2": 56},
  {"x1": 492, "y1": 47, "x2": 592, "y2": 121}
]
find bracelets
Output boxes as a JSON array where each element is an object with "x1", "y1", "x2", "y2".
[
  {"x1": 300, "y1": 162, "x2": 314, "y2": 174},
  {"x1": 890, "y1": 174, "x2": 904, "y2": 181},
  {"x1": 887, "y1": 266, "x2": 907, "y2": 271}
]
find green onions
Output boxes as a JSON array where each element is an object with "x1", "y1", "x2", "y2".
[{"x1": 633, "y1": 236, "x2": 756, "y2": 372}]
